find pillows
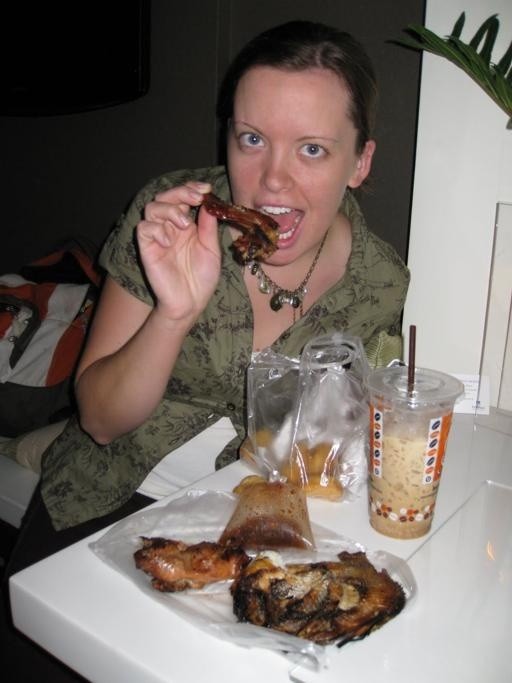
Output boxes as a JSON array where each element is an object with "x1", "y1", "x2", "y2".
[{"x1": 1, "y1": 416, "x2": 70, "y2": 473}]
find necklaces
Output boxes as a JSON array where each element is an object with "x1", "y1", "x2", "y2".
[{"x1": 246, "y1": 230, "x2": 329, "y2": 323}]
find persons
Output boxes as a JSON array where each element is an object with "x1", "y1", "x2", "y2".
[{"x1": 5, "y1": 21, "x2": 411, "y2": 577}]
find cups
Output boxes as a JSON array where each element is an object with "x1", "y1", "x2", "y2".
[{"x1": 364, "y1": 366, "x2": 464, "y2": 539}]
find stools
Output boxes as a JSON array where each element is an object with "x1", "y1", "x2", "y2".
[{"x1": 0, "y1": 432, "x2": 39, "y2": 530}]
